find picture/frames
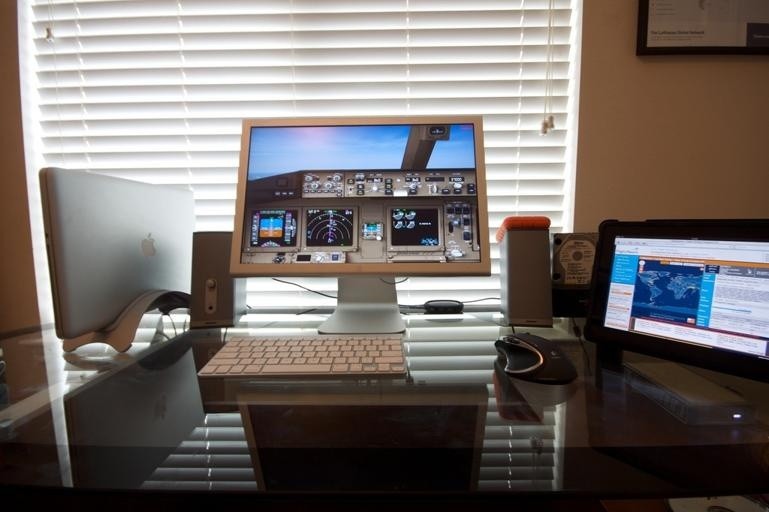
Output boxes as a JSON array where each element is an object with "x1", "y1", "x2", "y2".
[{"x1": 636, "y1": 0, "x2": 769, "y2": 56}]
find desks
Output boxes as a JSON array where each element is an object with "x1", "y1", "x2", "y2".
[{"x1": 0, "y1": 316, "x2": 768, "y2": 512}]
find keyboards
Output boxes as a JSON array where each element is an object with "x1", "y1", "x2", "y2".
[{"x1": 193, "y1": 331, "x2": 409, "y2": 378}]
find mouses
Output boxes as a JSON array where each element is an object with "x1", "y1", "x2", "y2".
[{"x1": 495, "y1": 328, "x2": 577, "y2": 386}]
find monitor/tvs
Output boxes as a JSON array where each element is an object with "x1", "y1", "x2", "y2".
[
  {"x1": 585, "y1": 217, "x2": 769, "y2": 384},
  {"x1": 229, "y1": 114, "x2": 492, "y2": 335},
  {"x1": 66, "y1": 348, "x2": 204, "y2": 485},
  {"x1": 39, "y1": 165, "x2": 191, "y2": 352},
  {"x1": 236, "y1": 391, "x2": 488, "y2": 494}
]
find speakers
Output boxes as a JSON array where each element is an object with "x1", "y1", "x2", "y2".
[
  {"x1": 191, "y1": 232, "x2": 247, "y2": 331},
  {"x1": 493, "y1": 228, "x2": 556, "y2": 327}
]
